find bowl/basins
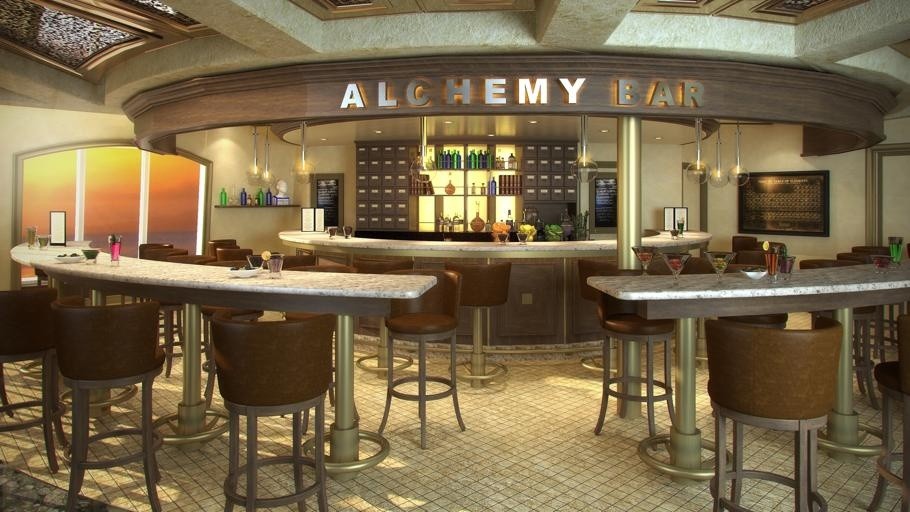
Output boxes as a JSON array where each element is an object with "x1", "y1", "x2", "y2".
[
  {"x1": 80, "y1": 250, "x2": 100, "y2": 259},
  {"x1": 741, "y1": 269, "x2": 766, "y2": 281}
]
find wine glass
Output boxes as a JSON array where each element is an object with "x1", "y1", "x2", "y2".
[
  {"x1": 704, "y1": 250, "x2": 739, "y2": 285},
  {"x1": 631, "y1": 244, "x2": 657, "y2": 282},
  {"x1": 657, "y1": 252, "x2": 691, "y2": 286}
]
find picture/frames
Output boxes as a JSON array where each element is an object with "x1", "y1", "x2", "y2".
[{"x1": 736, "y1": 170, "x2": 831, "y2": 237}]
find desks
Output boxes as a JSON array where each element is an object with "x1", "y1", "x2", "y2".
[
  {"x1": 585, "y1": 260, "x2": 910, "y2": 484},
  {"x1": 7, "y1": 238, "x2": 439, "y2": 473}
]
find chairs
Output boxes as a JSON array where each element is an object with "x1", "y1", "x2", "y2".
[
  {"x1": 1, "y1": 284, "x2": 68, "y2": 475},
  {"x1": 858, "y1": 312, "x2": 910, "y2": 511},
  {"x1": 705, "y1": 316, "x2": 846, "y2": 511},
  {"x1": 207, "y1": 310, "x2": 333, "y2": 510},
  {"x1": 48, "y1": 294, "x2": 167, "y2": 509}
]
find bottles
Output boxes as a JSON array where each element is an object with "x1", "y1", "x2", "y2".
[
  {"x1": 436, "y1": 149, "x2": 461, "y2": 169},
  {"x1": 470, "y1": 200, "x2": 485, "y2": 232},
  {"x1": 480, "y1": 182, "x2": 486, "y2": 196},
  {"x1": 444, "y1": 171, "x2": 456, "y2": 195},
  {"x1": 425, "y1": 148, "x2": 435, "y2": 170},
  {"x1": 506, "y1": 210, "x2": 513, "y2": 231},
  {"x1": 467, "y1": 148, "x2": 492, "y2": 168},
  {"x1": 219, "y1": 187, "x2": 272, "y2": 207},
  {"x1": 495, "y1": 152, "x2": 516, "y2": 169},
  {"x1": 436, "y1": 215, "x2": 463, "y2": 233},
  {"x1": 472, "y1": 183, "x2": 475, "y2": 195},
  {"x1": 491, "y1": 177, "x2": 497, "y2": 195}
]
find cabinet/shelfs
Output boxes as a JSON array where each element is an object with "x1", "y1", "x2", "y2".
[
  {"x1": 515, "y1": 142, "x2": 578, "y2": 202},
  {"x1": 420, "y1": 162, "x2": 518, "y2": 196},
  {"x1": 355, "y1": 142, "x2": 419, "y2": 228}
]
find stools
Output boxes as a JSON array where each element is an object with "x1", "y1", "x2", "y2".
[
  {"x1": 444, "y1": 256, "x2": 511, "y2": 389},
  {"x1": 133, "y1": 229, "x2": 465, "y2": 450},
  {"x1": 573, "y1": 235, "x2": 909, "y2": 450}
]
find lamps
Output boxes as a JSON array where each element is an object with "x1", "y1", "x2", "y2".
[
  {"x1": 247, "y1": 118, "x2": 311, "y2": 180},
  {"x1": 569, "y1": 114, "x2": 599, "y2": 183},
  {"x1": 683, "y1": 118, "x2": 748, "y2": 189}
]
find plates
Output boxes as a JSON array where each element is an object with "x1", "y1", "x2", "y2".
[
  {"x1": 53, "y1": 256, "x2": 82, "y2": 264},
  {"x1": 224, "y1": 267, "x2": 263, "y2": 279}
]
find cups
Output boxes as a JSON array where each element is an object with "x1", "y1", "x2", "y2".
[
  {"x1": 246, "y1": 252, "x2": 286, "y2": 278},
  {"x1": 870, "y1": 253, "x2": 891, "y2": 275},
  {"x1": 888, "y1": 236, "x2": 906, "y2": 270},
  {"x1": 764, "y1": 253, "x2": 779, "y2": 282},
  {"x1": 678, "y1": 219, "x2": 685, "y2": 237},
  {"x1": 495, "y1": 232, "x2": 507, "y2": 247},
  {"x1": 109, "y1": 234, "x2": 123, "y2": 261},
  {"x1": 776, "y1": 254, "x2": 796, "y2": 281},
  {"x1": 326, "y1": 225, "x2": 352, "y2": 241},
  {"x1": 516, "y1": 233, "x2": 527, "y2": 247},
  {"x1": 668, "y1": 229, "x2": 679, "y2": 241},
  {"x1": 36, "y1": 234, "x2": 49, "y2": 250}
]
